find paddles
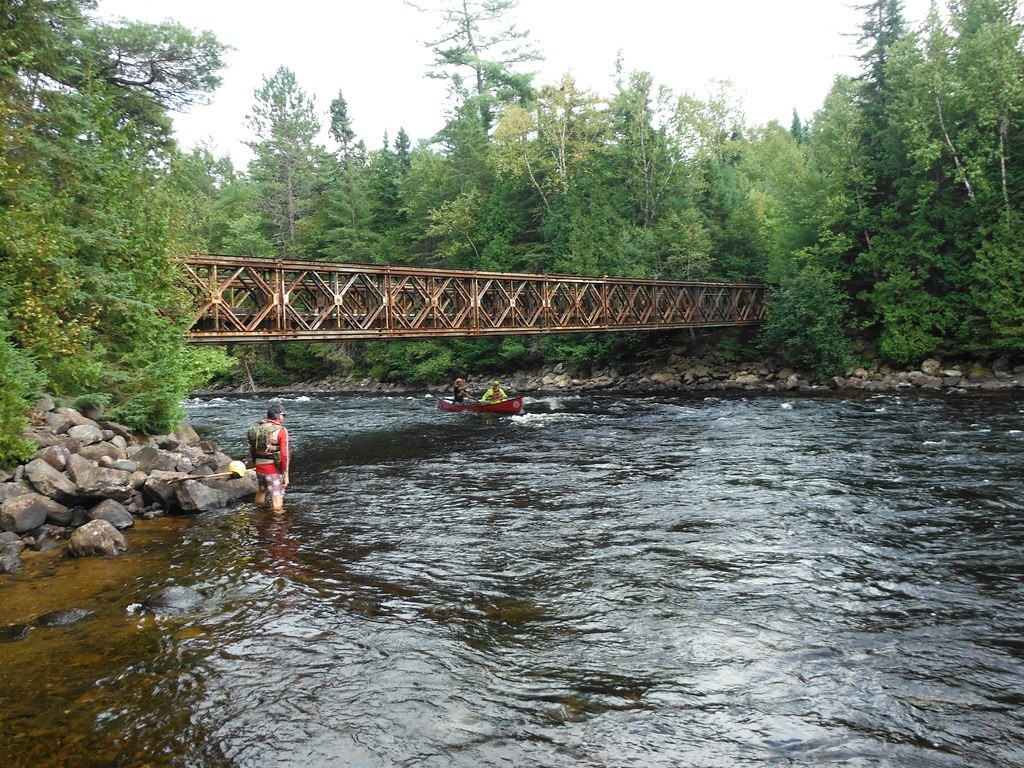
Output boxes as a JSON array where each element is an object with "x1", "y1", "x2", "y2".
[
  {"x1": 168, "y1": 470, "x2": 236, "y2": 487},
  {"x1": 471, "y1": 384, "x2": 480, "y2": 402}
]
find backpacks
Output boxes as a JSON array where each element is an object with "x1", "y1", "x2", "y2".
[{"x1": 248, "y1": 420, "x2": 280, "y2": 452}]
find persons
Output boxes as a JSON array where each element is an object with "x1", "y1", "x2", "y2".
[
  {"x1": 453, "y1": 378, "x2": 478, "y2": 406},
  {"x1": 248, "y1": 404, "x2": 289, "y2": 513},
  {"x1": 482, "y1": 381, "x2": 508, "y2": 404}
]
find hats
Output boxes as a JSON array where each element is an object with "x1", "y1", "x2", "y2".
[
  {"x1": 456, "y1": 378, "x2": 465, "y2": 382},
  {"x1": 493, "y1": 381, "x2": 499, "y2": 385}
]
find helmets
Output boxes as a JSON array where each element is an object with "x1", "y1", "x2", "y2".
[{"x1": 229, "y1": 461, "x2": 246, "y2": 478}]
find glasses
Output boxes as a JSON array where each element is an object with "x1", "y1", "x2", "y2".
[{"x1": 278, "y1": 414, "x2": 286, "y2": 417}]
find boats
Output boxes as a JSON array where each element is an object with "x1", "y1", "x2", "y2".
[{"x1": 437, "y1": 394, "x2": 524, "y2": 414}]
